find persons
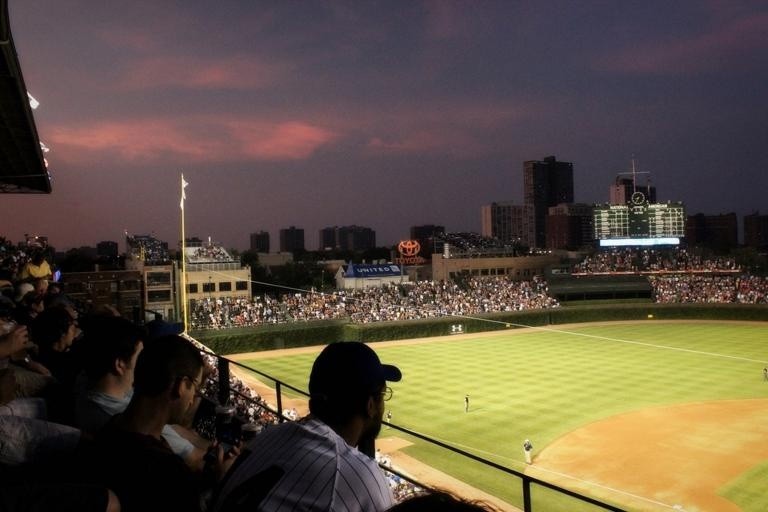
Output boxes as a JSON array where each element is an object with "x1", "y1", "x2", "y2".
[
  {"x1": 524, "y1": 440, "x2": 533, "y2": 464},
  {"x1": 1, "y1": 232, "x2": 401, "y2": 511},
  {"x1": 763, "y1": 367, "x2": 768, "y2": 381},
  {"x1": 567, "y1": 247, "x2": 768, "y2": 305},
  {"x1": 189, "y1": 276, "x2": 559, "y2": 331},
  {"x1": 389, "y1": 490, "x2": 487, "y2": 511},
  {"x1": 465, "y1": 394, "x2": 469, "y2": 412}
]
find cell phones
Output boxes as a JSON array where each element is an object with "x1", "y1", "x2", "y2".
[{"x1": 203, "y1": 434, "x2": 239, "y2": 464}]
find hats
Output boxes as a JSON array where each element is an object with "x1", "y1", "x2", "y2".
[
  {"x1": 310, "y1": 342, "x2": 401, "y2": 390},
  {"x1": 141, "y1": 320, "x2": 184, "y2": 342}
]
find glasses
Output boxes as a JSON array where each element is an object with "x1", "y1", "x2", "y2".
[
  {"x1": 189, "y1": 375, "x2": 208, "y2": 397},
  {"x1": 380, "y1": 387, "x2": 394, "y2": 401}
]
